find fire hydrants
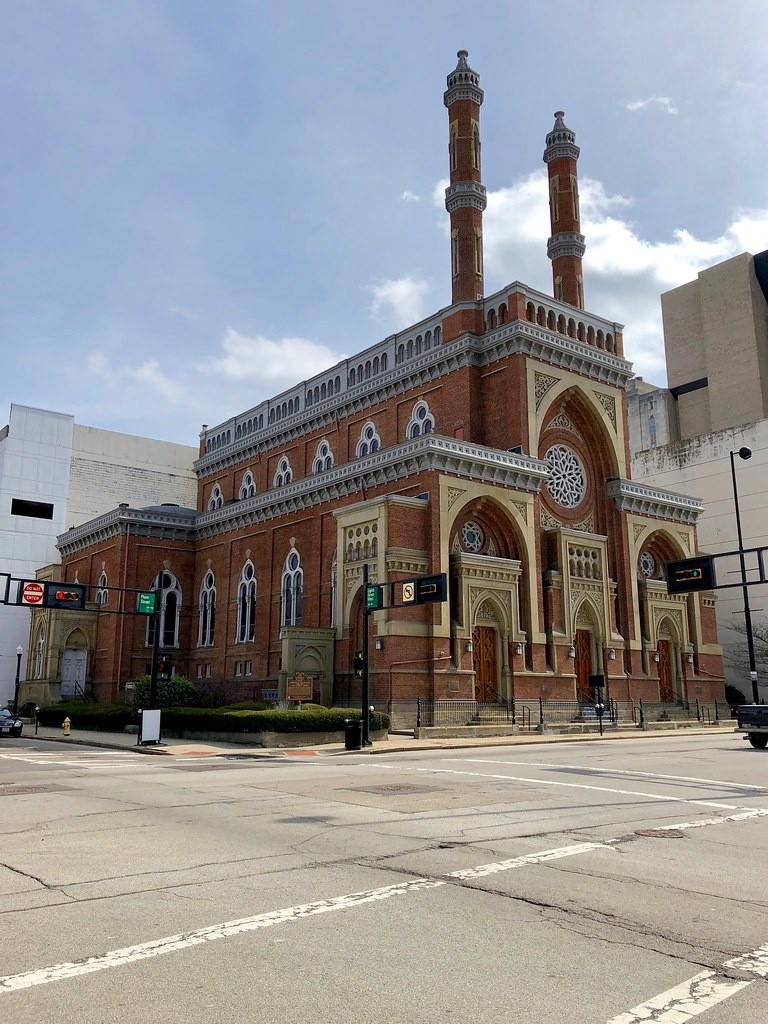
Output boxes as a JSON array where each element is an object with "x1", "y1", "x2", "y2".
[{"x1": 62, "y1": 716, "x2": 71, "y2": 736}]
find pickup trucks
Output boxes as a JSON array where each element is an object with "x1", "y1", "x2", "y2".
[{"x1": 729, "y1": 705, "x2": 768, "y2": 750}]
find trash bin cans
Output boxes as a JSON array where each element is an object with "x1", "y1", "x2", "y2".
[{"x1": 344, "y1": 718, "x2": 364, "y2": 751}]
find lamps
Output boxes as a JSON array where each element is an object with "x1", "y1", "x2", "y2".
[
  {"x1": 652, "y1": 653, "x2": 659, "y2": 662},
  {"x1": 608, "y1": 649, "x2": 615, "y2": 660},
  {"x1": 466, "y1": 639, "x2": 473, "y2": 652},
  {"x1": 375, "y1": 638, "x2": 382, "y2": 650},
  {"x1": 568, "y1": 647, "x2": 575, "y2": 658},
  {"x1": 686, "y1": 655, "x2": 693, "y2": 663},
  {"x1": 515, "y1": 643, "x2": 522, "y2": 655}
]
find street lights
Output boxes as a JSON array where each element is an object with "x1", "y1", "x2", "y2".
[
  {"x1": 13, "y1": 644, "x2": 23, "y2": 716},
  {"x1": 729, "y1": 445, "x2": 764, "y2": 704}
]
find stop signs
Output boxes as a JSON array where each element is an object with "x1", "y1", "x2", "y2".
[{"x1": 23, "y1": 584, "x2": 44, "y2": 604}]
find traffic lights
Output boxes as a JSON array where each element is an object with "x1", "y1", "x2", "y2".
[
  {"x1": 56, "y1": 591, "x2": 79, "y2": 600},
  {"x1": 158, "y1": 650, "x2": 167, "y2": 673},
  {"x1": 675, "y1": 569, "x2": 702, "y2": 581}
]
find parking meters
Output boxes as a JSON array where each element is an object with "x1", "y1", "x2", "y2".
[{"x1": 34, "y1": 706, "x2": 40, "y2": 735}]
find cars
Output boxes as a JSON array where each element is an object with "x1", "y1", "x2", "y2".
[{"x1": 0, "y1": 705, "x2": 24, "y2": 737}]
